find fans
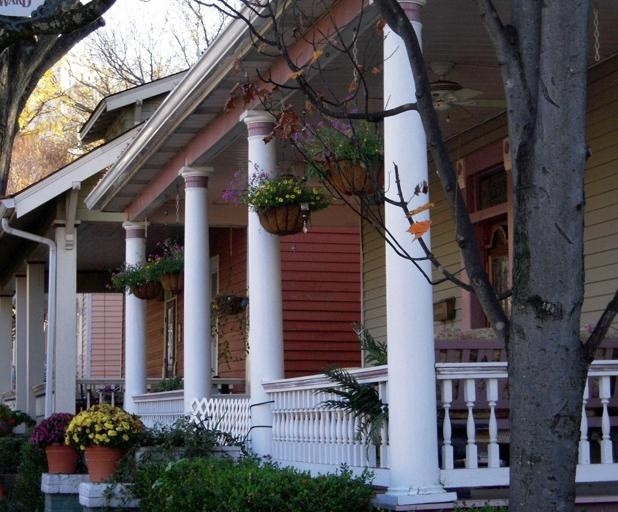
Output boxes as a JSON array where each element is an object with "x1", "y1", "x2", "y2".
[{"x1": 428, "y1": 61, "x2": 508, "y2": 112}]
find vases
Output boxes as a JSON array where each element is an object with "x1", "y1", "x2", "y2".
[{"x1": 44, "y1": 442, "x2": 124, "y2": 482}]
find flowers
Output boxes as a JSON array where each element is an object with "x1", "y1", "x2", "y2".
[
  {"x1": 100, "y1": 239, "x2": 185, "y2": 294},
  {"x1": 31, "y1": 404, "x2": 147, "y2": 451},
  {"x1": 223, "y1": 108, "x2": 383, "y2": 212}
]
